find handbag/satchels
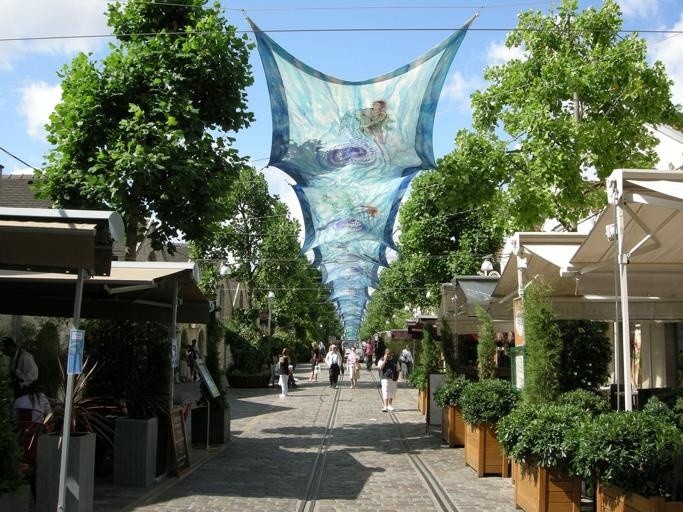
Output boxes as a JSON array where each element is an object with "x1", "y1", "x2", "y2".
[{"x1": 354, "y1": 364, "x2": 362, "y2": 371}]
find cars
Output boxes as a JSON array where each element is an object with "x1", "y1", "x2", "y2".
[{"x1": 343, "y1": 341, "x2": 364, "y2": 363}]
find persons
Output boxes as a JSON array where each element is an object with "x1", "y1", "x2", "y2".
[
  {"x1": 277, "y1": 347, "x2": 290, "y2": 398},
  {"x1": 375, "y1": 336, "x2": 387, "y2": 365},
  {"x1": 346, "y1": 346, "x2": 362, "y2": 388},
  {"x1": 0, "y1": 336, "x2": 53, "y2": 474},
  {"x1": 324, "y1": 344, "x2": 343, "y2": 390},
  {"x1": 308, "y1": 340, "x2": 326, "y2": 383},
  {"x1": 364, "y1": 338, "x2": 373, "y2": 372},
  {"x1": 377, "y1": 347, "x2": 401, "y2": 412},
  {"x1": 180, "y1": 344, "x2": 192, "y2": 383},
  {"x1": 335, "y1": 339, "x2": 345, "y2": 375},
  {"x1": 360, "y1": 339, "x2": 367, "y2": 352},
  {"x1": 188, "y1": 339, "x2": 199, "y2": 382}
]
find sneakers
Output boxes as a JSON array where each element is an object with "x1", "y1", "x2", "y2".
[{"x1": 382, "y1": 405, "x2": 395, "y2": 412}]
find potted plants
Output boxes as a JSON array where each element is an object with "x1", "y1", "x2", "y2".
[
  {"x1": 457, "y1": 378, "x2": 521, "y2": 478},
  {"x1": 568, "y1": 394, "x2": 683, "y2": 512},
  {"x1": 0, "y1": 354, "x2": 172, "y2": 512},
  {"x1": 493, "y1": 387, "x2": 613, "y2": 512},
  {"x1": 431, "y1": 375, "x2": 477, "y2": 449},
  {"x1": 408, "y1": 365, "x2": 449, "y2": 426}
]
